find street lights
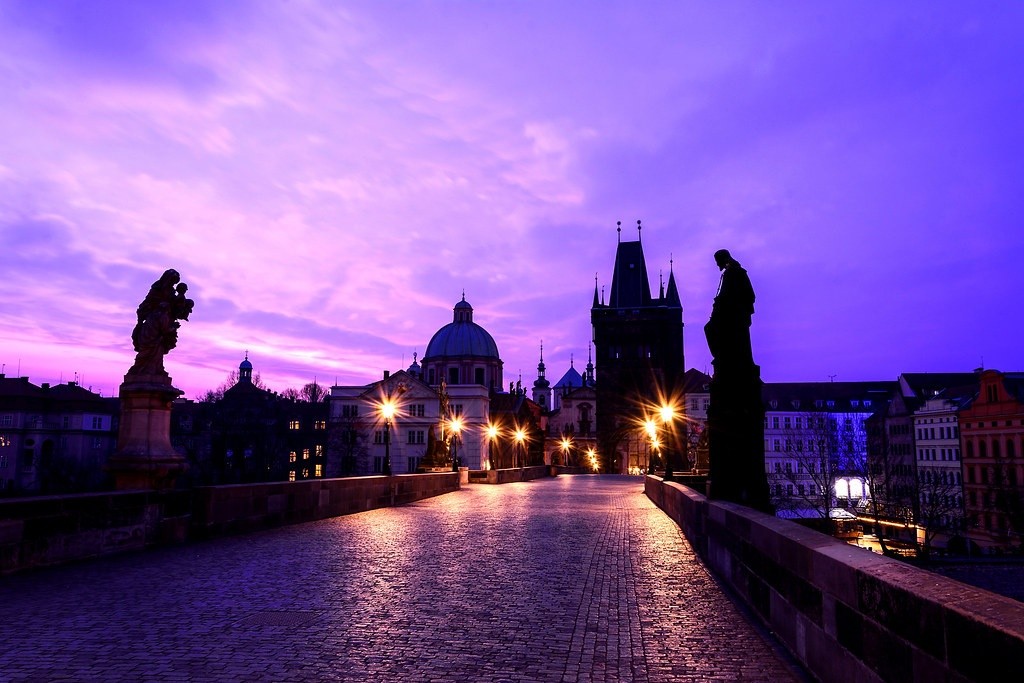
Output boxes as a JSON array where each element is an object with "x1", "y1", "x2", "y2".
[
  {"x1": 487, "y1": 426, "x2": 497, "y2": 469},
  {"x1": 450, "y1": 422, "x2": 460, "y2": 471},
  {"x1": 379, "y1": 404, "x2": 394, "y2": 476},
  {"x1": 662, "y1": 407, "x2": 674, "y2": 479},
  {"x1": 644, "y1": 422, "x2": 660, "y2": 472},
  {"x1": 563, "y1": 441, "x2": 568, "y2": 466},
  {"x1": 514, "y1": 432, "x2": 523, "y2": 467}
]
